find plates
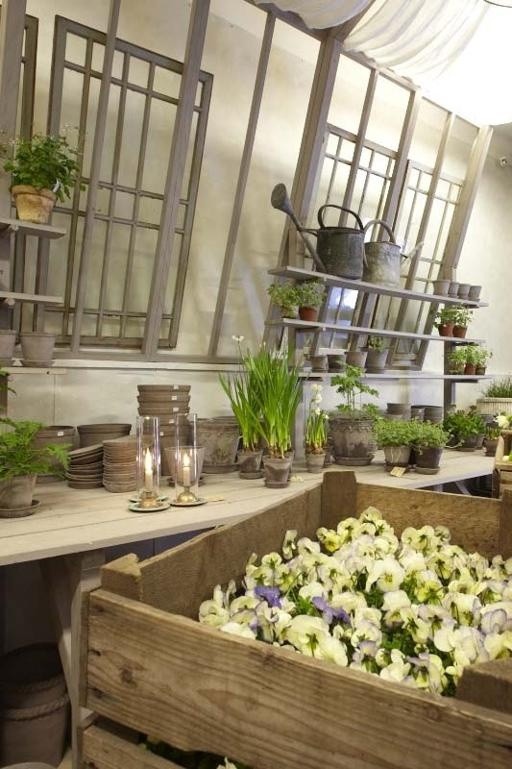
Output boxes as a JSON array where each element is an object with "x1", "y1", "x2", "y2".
[{"x1": 64, "y1": 435, "x2": 145, "y2": 494}]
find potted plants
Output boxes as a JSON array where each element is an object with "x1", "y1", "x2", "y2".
[
  {"x1": 0, "y1": 116, "x2": 90, "y2": 225},
  {"x1": 2, "y1": 415, "x2": 75, "y2": 517}
]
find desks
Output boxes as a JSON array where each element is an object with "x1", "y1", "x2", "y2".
[{"x1": 1, "y1": 446, "x2": 512, "y2": 717}]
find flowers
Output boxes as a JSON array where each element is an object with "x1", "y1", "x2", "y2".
[{"x1": 199, "y1": 500, "x2": 511, "y2": 699}]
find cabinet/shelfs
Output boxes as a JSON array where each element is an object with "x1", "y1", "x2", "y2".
[
  {"x1": 267, "y1": 262, "x2": 492, "y2": 445},
  {"x1": 1, "y1": 216, "x2": 72, "y2": 378}
]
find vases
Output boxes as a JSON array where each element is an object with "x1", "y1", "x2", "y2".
[
  {"x1": 25, "y1": 381, "x2": 242, "y2": 496},
  {"x1": 2, "y1": 643, "x2": 67, "y2": 709},
  {"x1": 2, "y1": 700, "x2": 71, "y2": 767}
]
